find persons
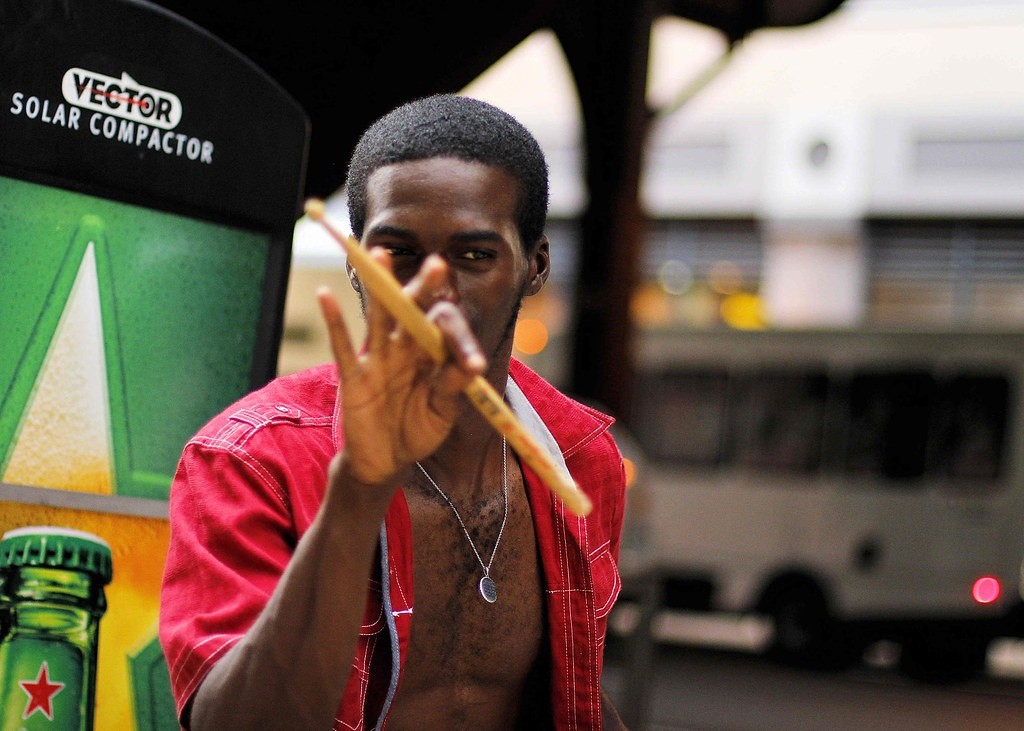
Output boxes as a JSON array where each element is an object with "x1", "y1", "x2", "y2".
[{"x1": 159, "y1": 95, "x2": 630, "y2": 731}]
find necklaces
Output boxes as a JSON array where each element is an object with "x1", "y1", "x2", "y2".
[{"x1": 414, "y1": 436, "x2": 508, "y2": 604}]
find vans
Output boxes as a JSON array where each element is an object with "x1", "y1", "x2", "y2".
[{"x1": 530, "y1": 319, "x2": 1023, "y2": 683}]
